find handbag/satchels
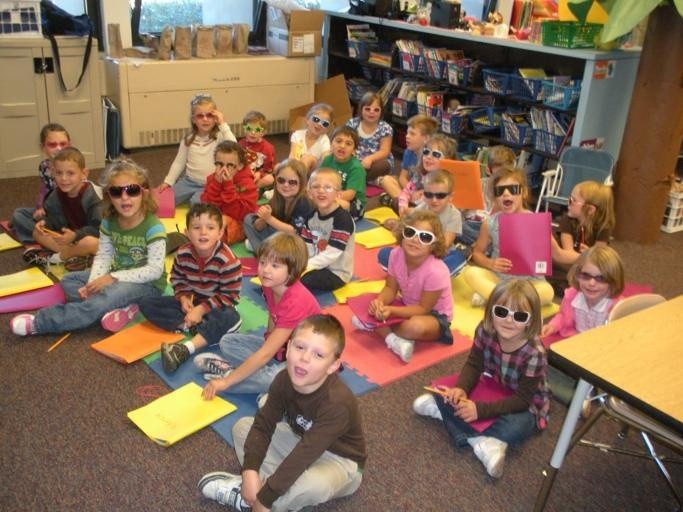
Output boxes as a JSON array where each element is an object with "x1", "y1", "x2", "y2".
[{"x1": 40, "y1": 0, "x2": 94, "y2": 41}]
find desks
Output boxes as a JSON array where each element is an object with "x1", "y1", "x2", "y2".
[{"x1": 534, "y1": 294, "x2": 683, "y2": 507}]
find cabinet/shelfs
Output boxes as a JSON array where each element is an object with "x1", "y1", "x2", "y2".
[
  {"x1": 321, "y1": 10, "x2": 642, "y2": 209},
  {"x1": 0, "y1": 36, "x2": 107, "y2": 178}
]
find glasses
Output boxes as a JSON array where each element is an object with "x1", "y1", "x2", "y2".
[
  {"x1": 578, "y1": 272, "x2": 609, "y2": 283},
  {"x1": 214, "y1": 161, "x2": 237, "y2": 172},
  {"x1": 106, "y1": 185, "x2": 146, "y2": 197},
  {"x1": 491, "y1": 305, "x2": 532, "y2": 326},
  {"x1": 567, "y1": 194, "x2": 591, "y2": 206},
  {"x1": 401, "y1": 221, "x2": 438, "y2": 246},
  {"x1": 491, "y1": 184, "x2": 523, "y2": 197},
  {"x1": 242, "y1": 123, "x2": 265, "y2": 135},
  {"x1": 42, "y1": 140, "x2": 69, "y2": 150},
  {"x1": 423, "y1": 192, "x2": 451, "y2": 201},
  {"x1": 309, "y1": 181, "x2": 340, "y2": 194},
  {"x1": 312, "y1": 116, "x2": 330, "y2": 130},
  {"x1": 274, "y1": 175, "x2": 297, "y2": 188},
  {"x1": 422, "y1": 146, "x2": 445, "y2": 160},
  {"x1": 194, "y1": 112, "x2": 216, "y2": 119}
]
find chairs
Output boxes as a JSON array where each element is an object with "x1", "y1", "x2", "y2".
[
  {"x1": 608, "y1": 293, "x2": 667, "y2": 437},
  {"x1": 535, "y1": 147, "x2": 614, "y2": 226}
]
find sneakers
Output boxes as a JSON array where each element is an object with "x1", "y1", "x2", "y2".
[
  {"x1": 8, "y1": 313, "x2": 37, "y2": 335},
  {"x1": 193, "y1": 352, "x2": 236, "y2": 382},
  {"x1": 351, "y1": 316, "x2": 508, "y2": 480},
  {"x1": 22, "y1": 248, "x2": 94, "y2": 271},
  {"x1": 101, "y1": 303, "x2": 139, "y2": 332},
  {"x1": 196, "y1": 471, "x2": 252, "y2": 512},
  {"x1": 161, "y1": 342, "x2": 190, "y2": 374}
]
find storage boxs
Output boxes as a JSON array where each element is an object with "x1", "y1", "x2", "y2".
[
  {"x1": 266, "y1": 4, "x2": 326, "y2": 58},
  {"x1": 289, "y1": 74, "x2": 353, "y2": 145}
]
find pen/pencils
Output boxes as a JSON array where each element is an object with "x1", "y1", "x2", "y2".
[
  {"x1": 43, "y1": 227, "x2": 60, "y2": 237},
  {"x1": 423, "y1": 385, "x2": 472, "y2": 404},
  {"x1": 48, "y1": 332, "x2": 72, "y2": 352},
  {"x1": 189, "y1": 295, "x2": 194, "y2": 310},
  {"x1": 380, "y1": 310, "x2": 386, "y2": 324}
]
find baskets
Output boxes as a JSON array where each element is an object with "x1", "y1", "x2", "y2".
[
  {"x1": 344, "y1": 34, "x2": 385, "y2": 61},
  {"x1": 334, "y1": 50, "x2": 582, "y2": 159},
  {"x1": 540, "y1": 21, "x2": 604, "y2": 50}
]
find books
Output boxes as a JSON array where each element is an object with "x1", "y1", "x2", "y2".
[
  {"x1": 427, "y1": 371, "x2": 515, "y2": 434},
  {"x1": 0, "y1": 266, "x2": 67, "y2": 315},
  {"x1": 345, "y1": 290, "x2": 415, "y2": 330},
  {"x1": 87, "y1": 316, "x2": 188, "y2": 365},
  {"x1": 124, "y1": 381, "x2": 237, "y2": 448},
  {"x1": 342, "y1": 20, "x2": 580, "y2": 154}
]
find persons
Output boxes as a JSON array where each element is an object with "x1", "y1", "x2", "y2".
[
  {"x1": 537, "y1": 242, "x2": 627, "y2": 421},
  {"x1": 411, "y1": 276, "x2": 550, "y2": 478},
  {"x1": 197, "y1": 314, "x2": 368, "y2": 512},
  {"x1": 352, "y1": 207, "x2": 454, "y2": 365},
  {"x1": 191, "y1": 230, "x2": 327, "y2": 411},
  {"x1": 137, "y1": 201, "x2": 244, "y2": 373},
  {"x1": 3, "y1": 93, "x2": 617, "y2": 308},
  {"x1": 7, "y1": 151, "x2": 168, "y2": 338}
]
